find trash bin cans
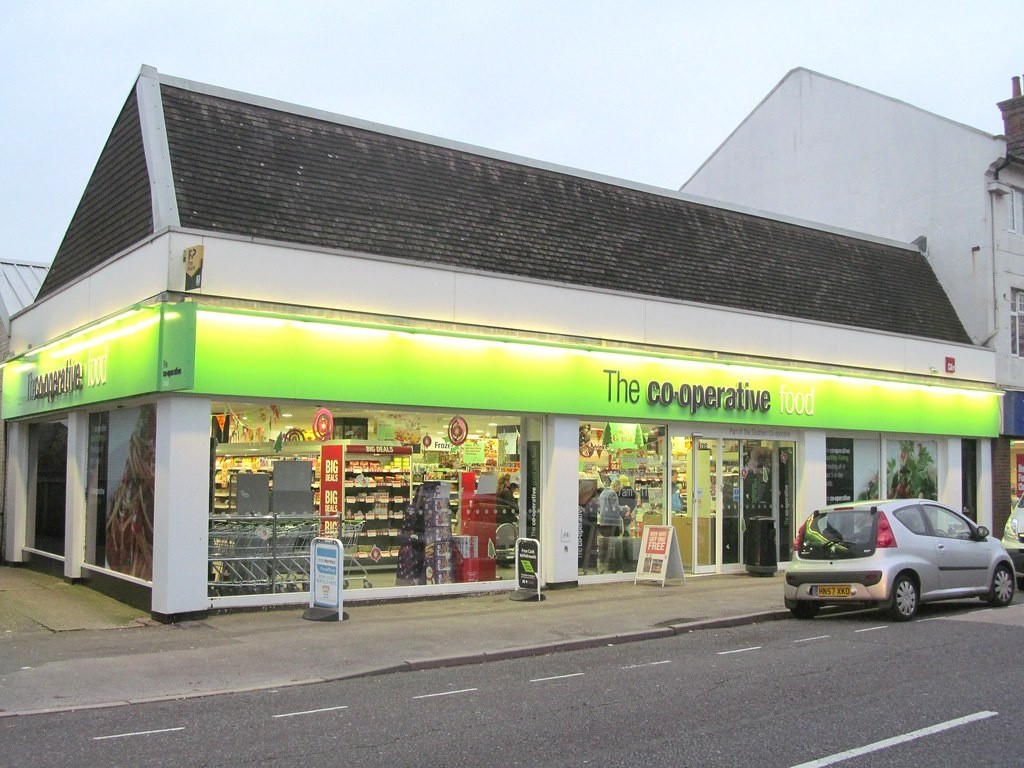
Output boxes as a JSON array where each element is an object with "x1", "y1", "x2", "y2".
[{"x1": 746, "y1": 516, "x2": 778, "y2": 577}]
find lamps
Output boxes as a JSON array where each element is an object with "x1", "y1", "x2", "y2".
[
  {"x1": 708, "y1": 352, "x2": 718, "y2": 359},
  {"x1": 929, "y1": 363, "x2": 938, "y2": 374}
]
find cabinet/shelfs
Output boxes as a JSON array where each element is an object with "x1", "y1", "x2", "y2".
[{"x1": 214, "y1": 443, "x2": 519, "y2": 576}]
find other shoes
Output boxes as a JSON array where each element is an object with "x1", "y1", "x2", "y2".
[{"x1": 500, "y1": 564, "x2": 513, "y2": 569}]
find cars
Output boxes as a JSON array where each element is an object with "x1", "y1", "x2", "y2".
[
  {"x1": 1001, "y1": 491, "x2": 1024, "y2": 591},
  {"x1": 783, "y1": 498, "x2": 1017, "y2": 622}
]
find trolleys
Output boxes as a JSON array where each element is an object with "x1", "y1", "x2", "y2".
[{"x1": 208, "y1": 518, "x2": 373, "y2": 597}]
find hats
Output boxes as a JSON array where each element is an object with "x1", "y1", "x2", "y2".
[{"x1": 620, "y1": 475, "x2": 631, "y2": 486}]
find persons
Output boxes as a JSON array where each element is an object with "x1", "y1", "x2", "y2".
[
  {"x1": 497, "y1": 471, "x2": 519, "y2": 523},
  {"x1": 672, "y1": 469, "x2": 683, "y2": 513},
  {"x1": 578, "y1": 472, "x2": 637, "y2": 575}
]
